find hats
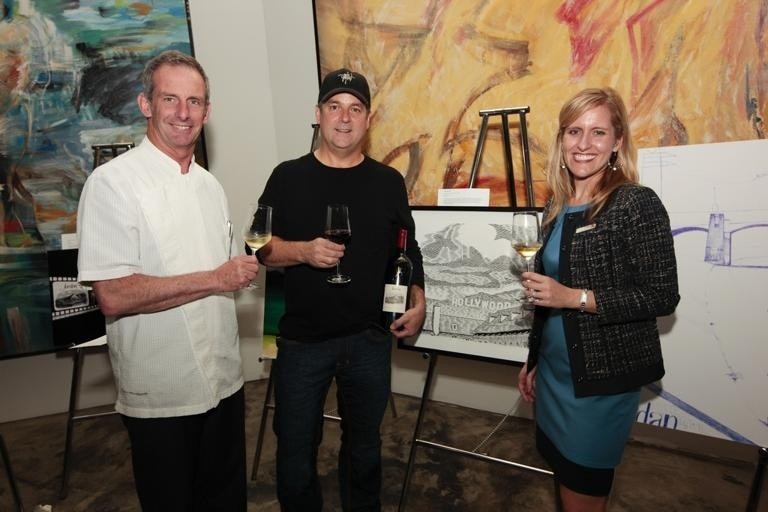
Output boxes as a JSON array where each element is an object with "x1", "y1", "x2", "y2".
[{"x1": 318, "y1": 69, "x2": 370, "y2": 108}]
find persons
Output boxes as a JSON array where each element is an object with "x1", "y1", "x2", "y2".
[
  {"x1": 517, "y1": 85, "x2": 680, "y2": 511},
  {"x1": 75, "y1": 48, "x2": 261, "y2": 510},
  {"x1": 244, "y1": 68, "x2": 427, "y2": 512}
]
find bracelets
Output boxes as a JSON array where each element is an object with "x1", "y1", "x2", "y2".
[{"x1": 579, "y1": 288, "x2": 587, "y2": 312}]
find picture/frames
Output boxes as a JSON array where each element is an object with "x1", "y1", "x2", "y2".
[{"x1": 397, "y1": 204, "x2": 546, "y2": 367}]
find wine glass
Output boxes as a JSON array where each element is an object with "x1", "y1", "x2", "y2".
[
  {"x1": 240, "y1": 204, "x2": 274, "y2": 292},
  {"x1": 511, "y1": 211, "x2": 543, "y2": 304},
  {"x1": 321, "y1": 204, "x2": 353, "y2": 285}
]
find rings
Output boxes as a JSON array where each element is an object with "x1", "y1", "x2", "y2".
[{"x1": 532, "y1": 292, "x2": 536, "y2": 297}]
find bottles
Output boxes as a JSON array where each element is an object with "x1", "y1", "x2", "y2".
[{"x1": 381, "y1": 226, "x2": 413, "y2": 338}]
